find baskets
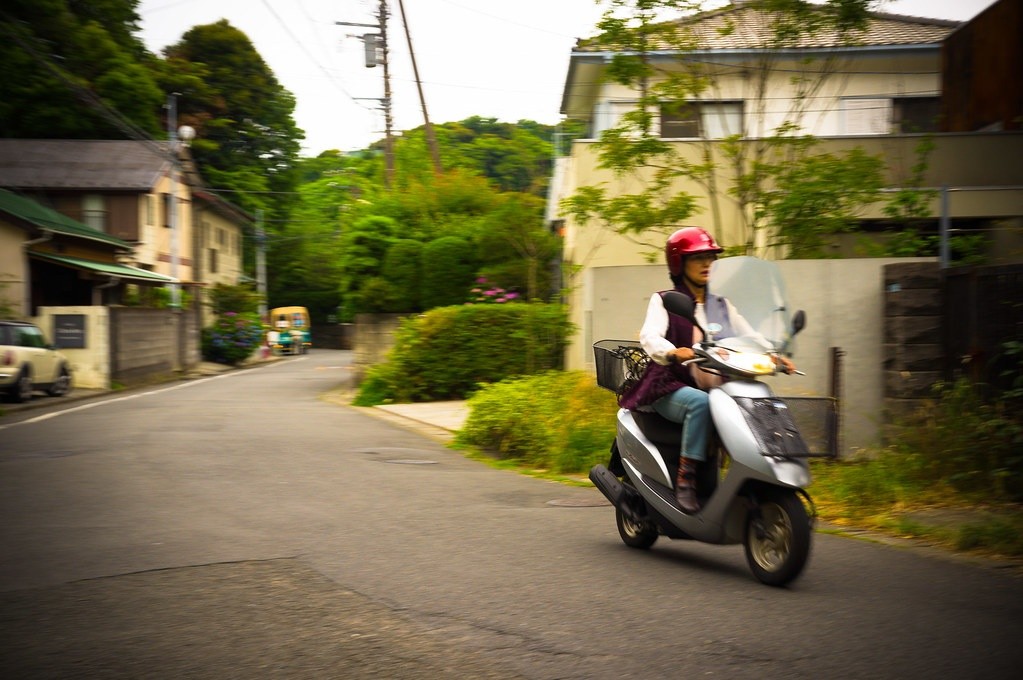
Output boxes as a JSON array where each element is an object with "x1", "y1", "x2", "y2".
[{"x1": 593, "y1": 338, "x2": 652, "y2": 395}]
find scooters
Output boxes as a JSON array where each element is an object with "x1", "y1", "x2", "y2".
[{"x1": 583, "y1": 309, "x2": 840, "y2": 588}]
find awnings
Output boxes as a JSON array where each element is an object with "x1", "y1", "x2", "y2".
[
  {"x1": 0, "y1": 189, "x2": 136, "y2": 254},
  {"x1": 29, "y1": 250, "x2": 180, "y2": 289}
]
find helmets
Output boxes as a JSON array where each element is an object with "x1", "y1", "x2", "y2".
[{"x1": 666, "y1": 226, "x2": 723, "y2": 276}]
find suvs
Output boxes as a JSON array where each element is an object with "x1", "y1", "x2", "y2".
[{"x1": 0, "y1": 320, "x2": 72, "y2": 404}]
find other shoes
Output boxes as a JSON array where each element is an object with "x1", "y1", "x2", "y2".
[{"x1": 676, "y1": 481, "x2": 700, "y2": 514}]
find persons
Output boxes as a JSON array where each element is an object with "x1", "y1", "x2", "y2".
[{"x1": 617, "y1": 227, "x2": 797, "y2": 515}]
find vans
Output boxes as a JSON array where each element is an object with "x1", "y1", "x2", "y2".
[{"x1": 267, "y1": 306, "x2": 311, "y2": 355}]
